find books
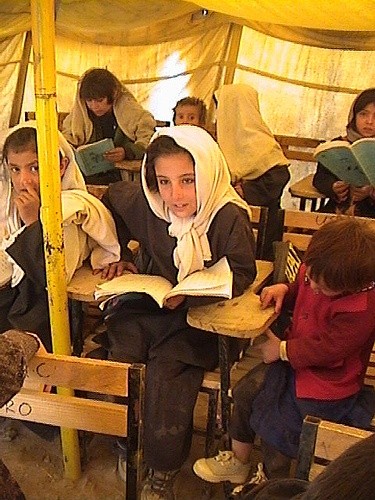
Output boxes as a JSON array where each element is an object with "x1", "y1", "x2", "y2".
[
  {"x1": 313, "y1": 137, "x2": 375, "y2": 187},
  {"x1": 70, "y1": 138, "x2": 116, "y2": 176},
  {"x1": 94, "y1": 256, "x2": 233, "y2": 311}
]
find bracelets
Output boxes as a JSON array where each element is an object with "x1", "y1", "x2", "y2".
[{"x1": 280, "y1": 341, "x2": 289, "y2": 361}]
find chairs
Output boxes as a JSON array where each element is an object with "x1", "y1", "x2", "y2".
[{"x1": 0, "y1": 111, "x2": 375, "y2": 500}]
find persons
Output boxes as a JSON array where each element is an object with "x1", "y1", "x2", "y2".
[
  {"x1": 312, "y1": 88, "x2": 375, "y2": 219},
  {"x1": 212, "y1": 83, "x2": 291, "y2": 214},
  {"x1": 192, "y1": 217, "x2": 375, "y2": 485},
  {"x1": 92, "y1": 123, "x2": 257, "y2": 500},
  {"x1": 172, "y1": 97, "x2": 207, "y2": 127},
  {"x1": 0, "y1": 120, "x2": 91, "y2": 353},
  {"x1": 62, "y1": 69, "x2": 156, "y2": 186}
]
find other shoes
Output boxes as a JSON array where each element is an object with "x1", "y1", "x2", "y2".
[
  {"x1": 193, "y1": 450, "x2": 251, "y2": 485},
  {"x1": 140, "y1": 467, "x2": 179, "y2": 500},
  {"x1": 114, "y1": 438, "x2": 146, "y2": 482},
  {"x1": 232, "y1": 462, "x2": 288, "y2": 500}
]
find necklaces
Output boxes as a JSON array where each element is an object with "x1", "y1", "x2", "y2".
[{"x1": 357, "y1": 281, "x2": 375, "y2": 292}]
